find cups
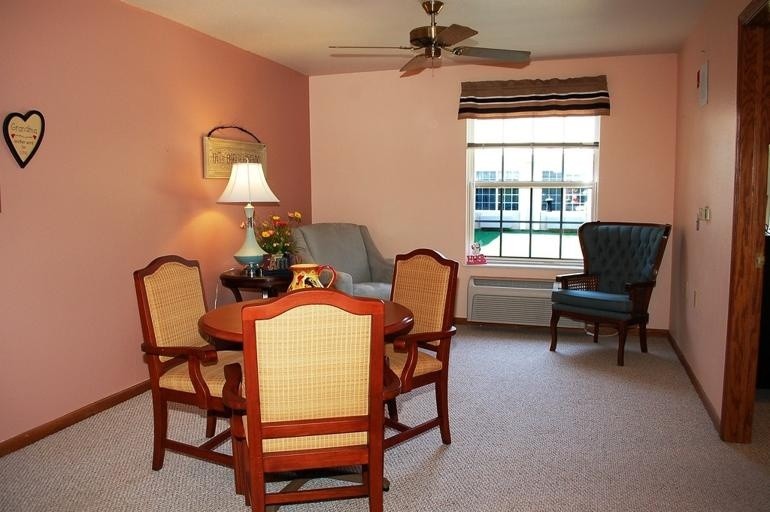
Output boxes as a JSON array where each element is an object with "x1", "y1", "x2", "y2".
[{"x1": 241, "y1": 267, "x2": 264, "y2": 278}]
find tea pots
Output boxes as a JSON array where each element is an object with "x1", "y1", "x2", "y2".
[{"x1": 286, "y1": 262, "x2": 338, "y2": 292}]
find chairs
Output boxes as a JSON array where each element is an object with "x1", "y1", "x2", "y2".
[
  {"x1": 293, "y1": 223, "x2": 397, "y2": 300},
  {"x1": 371, "y1": 244, "x2": 459, "y2": 453},
  {"x1": 231, "y1": 298, "x2": 393, "y2": 512},
  {"x1": 543, "y1": 217, "x2": 672, "y2": 368},
  {"x1": 130, "y1": 254, "x2": 245, "y2": 472}
]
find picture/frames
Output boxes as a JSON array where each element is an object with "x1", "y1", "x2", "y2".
[{"x1": 1, "y1": 108, "x2": 48, "y2": 169}]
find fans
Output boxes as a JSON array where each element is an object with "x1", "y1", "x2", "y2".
[{"x1": 325, "y1": 0, "x2": 534, "y2": 72}]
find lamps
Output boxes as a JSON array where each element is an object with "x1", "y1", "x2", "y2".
[{"x1": 217, "y1": 162, "x2": 281, "y2": 266}]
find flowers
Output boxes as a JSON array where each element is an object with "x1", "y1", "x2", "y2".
[{"x1": 239, "y1": 211, "x2": 302, "y2": 253}]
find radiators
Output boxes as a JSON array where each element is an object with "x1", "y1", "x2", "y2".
[{"x1": 465, "y1": 275, "x2": 589, "y2": 331}]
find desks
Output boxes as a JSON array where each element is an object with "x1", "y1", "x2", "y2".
[
  {"x1": 197, "y1": 300, "x2": 414, "y2": 512},
  {"x1": 219, "y1": 267, "x2": 288, "y2": 302}
]
